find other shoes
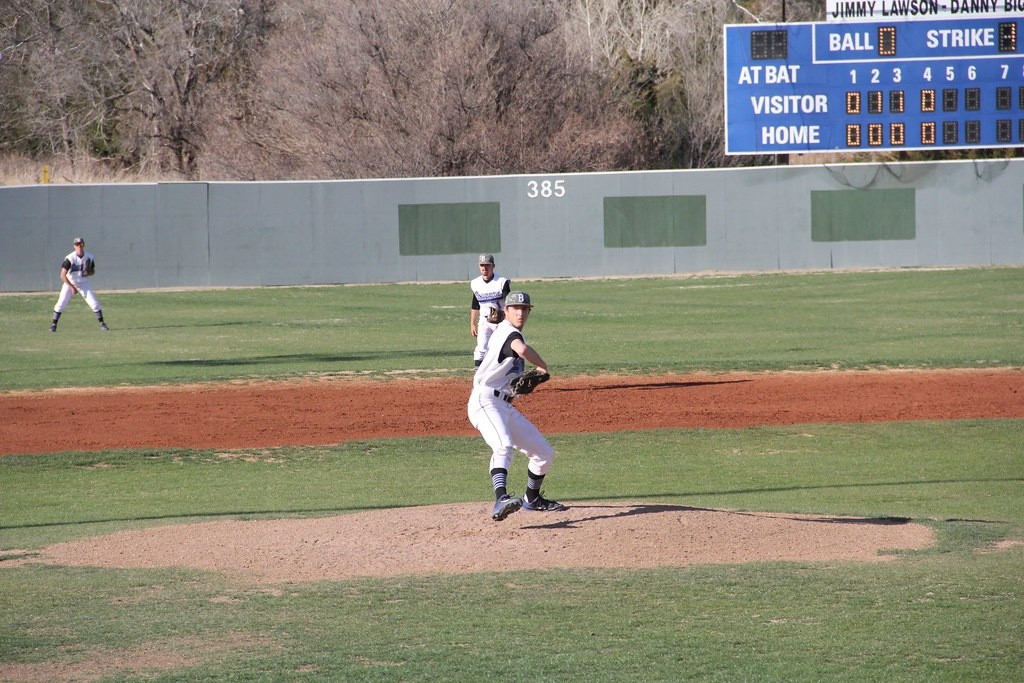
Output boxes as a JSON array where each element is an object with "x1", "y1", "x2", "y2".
[
  {"x1": 101, "y1": 326, "x2": 109, "y2": 330},
  {"x1": 49, "y1": 324, "x2": 56, "y2": 331}
]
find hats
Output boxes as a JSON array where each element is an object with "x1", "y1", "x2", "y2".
[
  {"x1": 479, "y1": 254, "x2": 494, "y2": 264},
  {"x1": 73, "y1": 238, "x2": 83, "y2": 244},
  {"x1": 505, "y1": 291, "x2": 534, "y2": 307}
]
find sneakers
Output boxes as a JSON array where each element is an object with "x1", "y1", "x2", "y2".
[
  {"x1": 491, "y1": 491, "x2": 523, "y2": 521},
  {"x1": 522, "y1": 494, "x2": 562, "y2": 511}
]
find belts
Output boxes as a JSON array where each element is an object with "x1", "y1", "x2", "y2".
[{"x1": 494, "y1": 389, "x2": 513, "y2": 402}]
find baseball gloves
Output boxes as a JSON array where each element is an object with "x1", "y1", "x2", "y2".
[
  {"x1": 82, "y1": 257, "x2": 95, "y2": 277},
  {"x1": 486, "y1": 306, "x2": 505, "y2": 324},
  {"x1": 509, "y1": 368, "x2": 552, "y2": 398}
]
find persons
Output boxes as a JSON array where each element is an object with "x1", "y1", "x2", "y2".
[
  {"x1": 470, "y1": 254, "x2": 511, "y2": 373},
  {"x1": 49, "y1": 238, "x2": 109, "y2": 332},
  {"x1": 467, "y1": 291, "x2": 565, "y2": 522}
]
